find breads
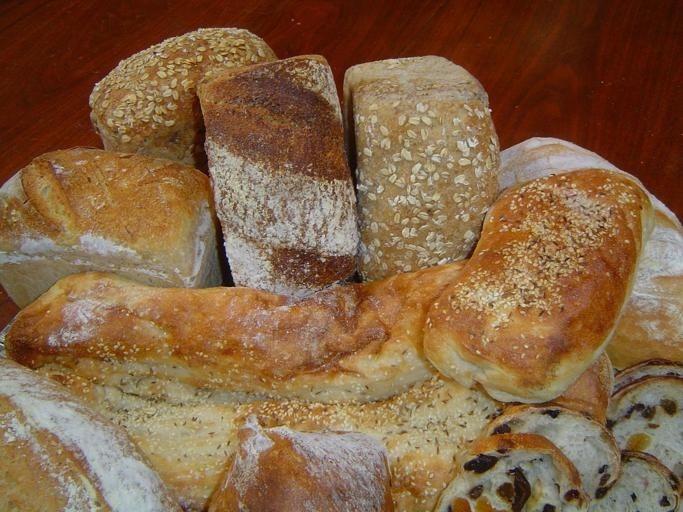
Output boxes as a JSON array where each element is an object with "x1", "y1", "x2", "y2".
[{"x1": 0, "y1": 28, "x2": 683, "y2": 512}]
